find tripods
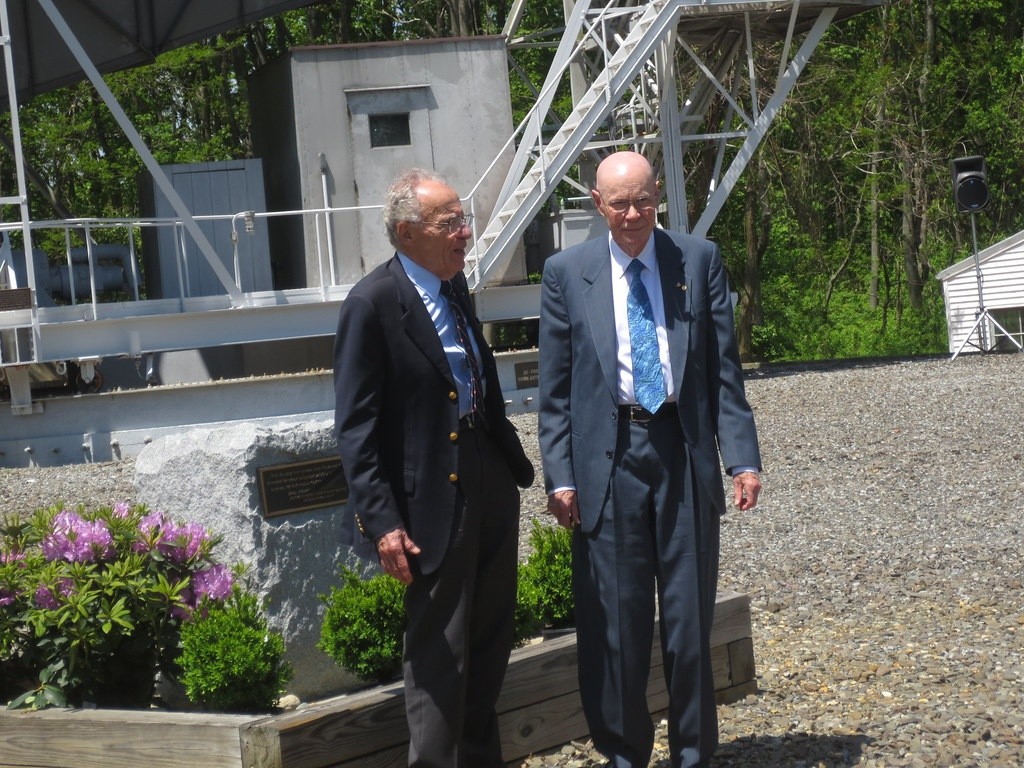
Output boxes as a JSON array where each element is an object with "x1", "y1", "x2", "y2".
[{"x1": 947, "y1": 213, "x2": 1024, "y2": 364}]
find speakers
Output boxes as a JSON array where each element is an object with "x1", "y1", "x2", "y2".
[{"x1": 949, "y1": 154, "x2": 991, "y2": 214}]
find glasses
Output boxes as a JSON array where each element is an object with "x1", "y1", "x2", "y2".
[
  {"x1": 597, "y1": 193, "x2": 658, "y2": 213},
  {"x1": 408, "y1": 213, "x2": 474, "y2": 233}
]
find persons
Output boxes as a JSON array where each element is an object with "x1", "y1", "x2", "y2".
[
  {"x1": 333, "y1": 170, "x2": 535, "y2": 768},
  {"x1": 538, "y1": 151, "x2": 763, "y2": 768}
]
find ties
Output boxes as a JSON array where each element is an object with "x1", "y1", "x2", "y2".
[
  {"x1": 627, "y1": 258, "x2": 668, "y2": 415},
  {"x1": 441, "y1": 281, "x2": 486, "y2": 425}
]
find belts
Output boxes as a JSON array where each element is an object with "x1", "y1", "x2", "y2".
[
  {"x1": 458, "y1": 411, "x2": 489, "y2": 433},
  {"x1": 618, "y1": 405, "x2": 681, "y2": 423}
]
